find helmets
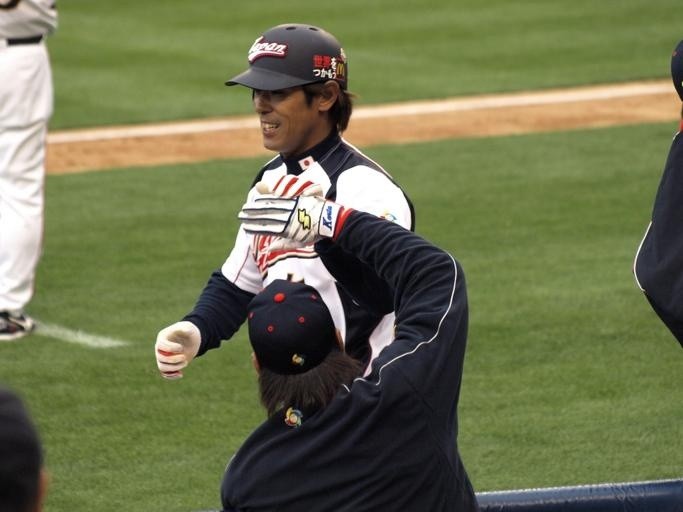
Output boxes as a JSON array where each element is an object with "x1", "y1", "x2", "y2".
[{"x1": 224, "y1": 23, "x2": 348, "y2": 93}]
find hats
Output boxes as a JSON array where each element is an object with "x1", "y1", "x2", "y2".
[{"x1": 245, "y1": 279, "x2": 334, "y2": 375}]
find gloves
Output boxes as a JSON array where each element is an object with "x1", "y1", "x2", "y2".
[
  {"x1": 236, "y1": 173, "x2": 344, "y2": 253},
  {"x1": 154, "y1": 321, "x2": 202, "y2": 382}
]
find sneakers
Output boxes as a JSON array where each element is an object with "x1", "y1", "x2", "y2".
[{"x1": 1, "y1": 310, "x2": 36, "y2": 342}]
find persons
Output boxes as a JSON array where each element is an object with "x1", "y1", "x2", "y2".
[
  {"x1": 0, "y1": 0, "x2": 57, "y2": 346},
  {"x1": 0, "y1": 383, "x2": 51, "y2": 512},
  {"x1": 218, "y1": 192, "x2": 480, "y2": 508},
  {"x1": 630, "y1": 39, "x2": 681, "y2": 350},
  {"x1": 152, "y1": 23, "x2": 418, "y2": 384}
]
